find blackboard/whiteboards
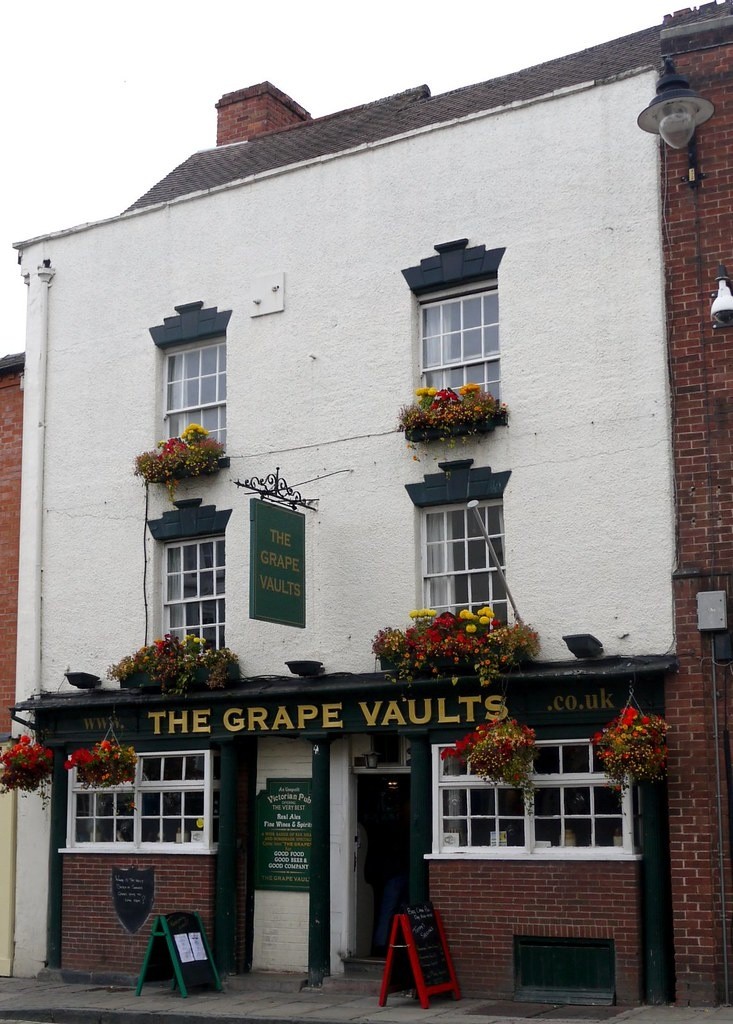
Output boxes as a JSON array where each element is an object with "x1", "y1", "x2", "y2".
[
  {"x1": 160, "y1": 912, "x2": 224, "y2": 998},
  {"x1": 398, "y1": 903, "x2": 461, "y2": 1008}
]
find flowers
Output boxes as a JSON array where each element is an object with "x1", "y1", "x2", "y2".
[
  {"x1": 372, "y1": 606, "x2": 538, "y2": 687},
  {"x1": 396, "y1": 382, "x2": 509, "y2": 462},
  {"x1": 107, "y1": 633, "x2": 239, "y2": 700},
  {"x1": 65, "y1": 715, "x2": 139, "y2": 814},
  {"x1": 0, "y1": 712, "x2": 53, "y2": 810},
  {"x1": 590, "y1": 678, "x2": 672, "y2": 807},
  {"x1": 442, "y1": 680, "x2": 541, "y2": 817},
  {"x1": 136, "y1": 424, "x2": 225, "y2": 502}
]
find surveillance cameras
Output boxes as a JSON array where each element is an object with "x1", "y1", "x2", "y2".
[{"x1": 711, "y1": 279, "x2": 733, "y2": 324}]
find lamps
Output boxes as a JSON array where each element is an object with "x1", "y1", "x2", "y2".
[
  {"x1": 637, "y1": 53, "x2": 715, "y2": 150},
  {"x1": 286, "y1": 660, "x2": 327, "y2": 679},
  {"x1": 66, "y1": 672, "x2": 101, "y2": 692},
  {"x1": 563, "y1": 632, "x2": 605, "y2": 661}
]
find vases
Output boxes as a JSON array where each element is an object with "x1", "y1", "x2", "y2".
[
  {"x1": 379, "y1": 652, "x2": 398, "y2": 670},
  {"x1": 119, "y1": 669, "x2": 232, "y2": 689}
]
